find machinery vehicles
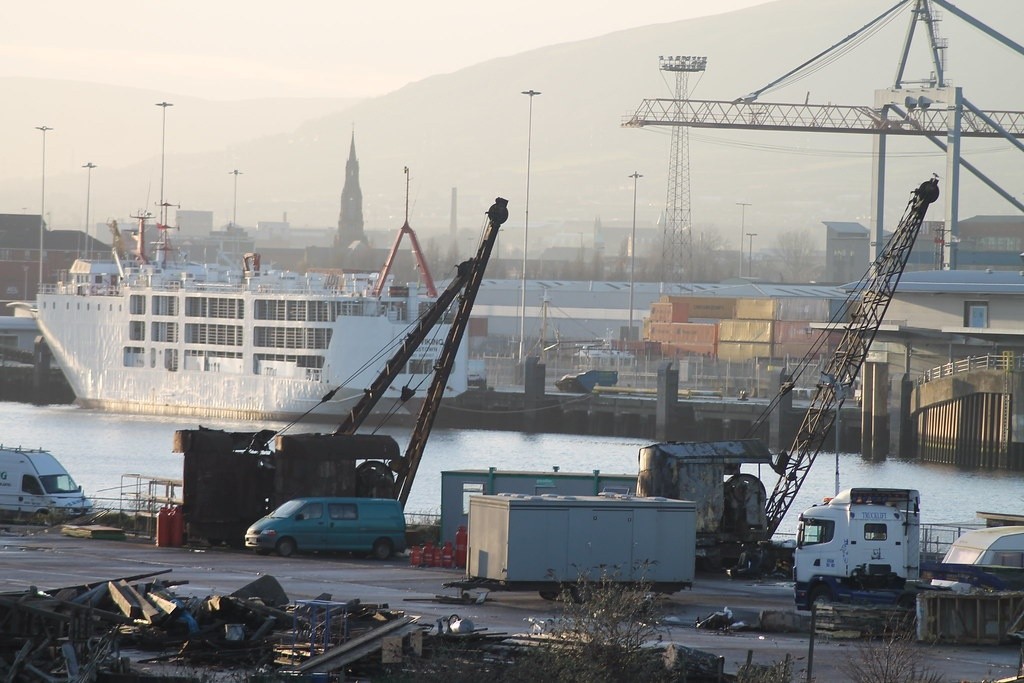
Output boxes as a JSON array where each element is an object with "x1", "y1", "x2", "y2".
[
  {"x1": 637, "y1": 172, "x2": 942, "y2": 563},
  {"x1": 821, "y1": 424, "x2": 825, "y2": 430},
  {"x1": 173, "y1": 198, "x2": 509, "y2": 547}
]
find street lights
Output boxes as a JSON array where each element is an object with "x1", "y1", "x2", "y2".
[
  {"x1": 229, "y1": 169, "x2": 242, "y2": 229},
  {"x1": 519, "y1": 90, "x2": 542, "y2": 363},
  {"x1": 156, "y1": 101, "x2": 173, "y2": 224},
  {"x1": 82, "y1": 162, "x2": 97, "y2": 257},
  {"x1": 746, "y1": 233, "x2": 757, "y2": 277},
  {"x1": 737, "y1": 202, "x2": 752, "y2": 275},
  {"x1": 36, "y1": 126, "x2": 53, "y2": 291},
  {"x1": 629, "y1": 170, "x2": 643, "y2": 339}
]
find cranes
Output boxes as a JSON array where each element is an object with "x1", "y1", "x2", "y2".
[{"x1": 622, "y1": 0, "x2": 1024, "y2": 296}]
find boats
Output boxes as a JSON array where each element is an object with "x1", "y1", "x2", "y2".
[{"x1": 32, "y1": 165, "x2": 467, "y2": 421}]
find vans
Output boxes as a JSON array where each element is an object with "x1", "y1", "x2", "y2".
[
  {"x1": 244, "y1": 498, "x2": 407, "y2": 562},
  {"x1": 942, "y1": 525, "x2": 1024, "y2": 567}
]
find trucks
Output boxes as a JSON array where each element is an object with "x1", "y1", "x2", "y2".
[
  {"x1": 467, "y1": 360, "x2": 486, "y2": 388},
  {"x1": 0, "y1": 445, "x2": 93, "y2": 527},
  {"x1": 793, "y1": 486, "x2": 1008, "y2": 614}
]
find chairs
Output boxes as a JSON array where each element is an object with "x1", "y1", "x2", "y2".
[{"x1": 873, "y1": 531, "x2": 885, "y2": 540}]
